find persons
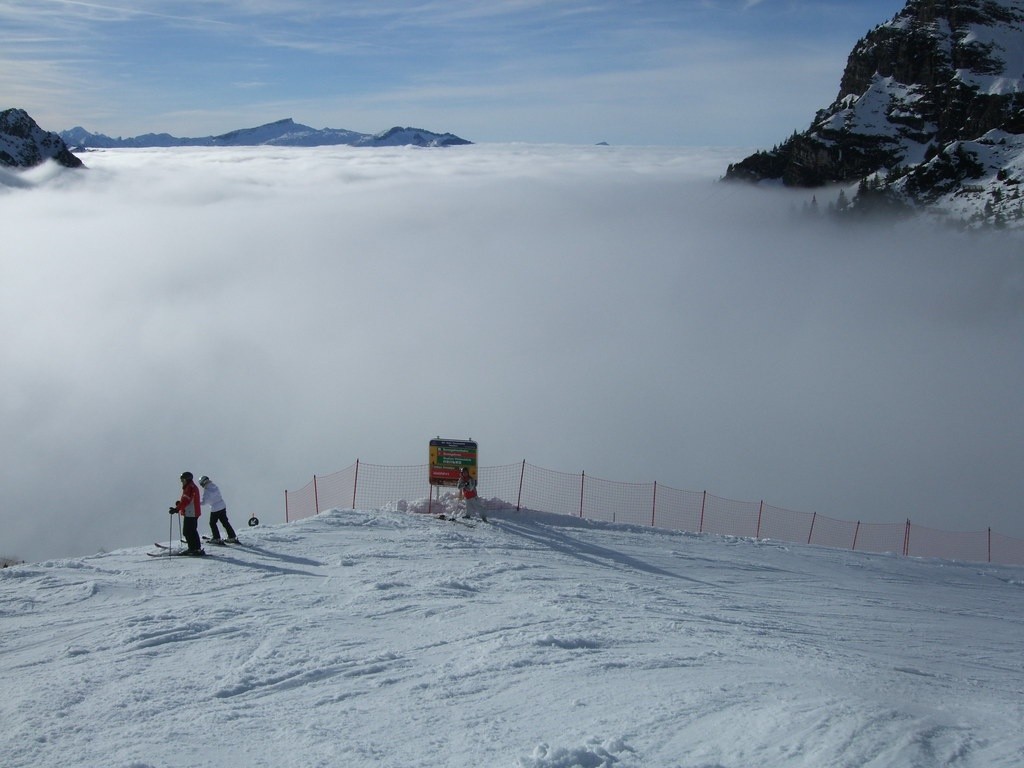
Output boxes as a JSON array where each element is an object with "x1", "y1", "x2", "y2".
[
  {"x1": 457, "y1": 468, "x2": 487, "y2": 523},
  {"x1": 198, "y1": 475, "x2": 236, "y2": 544},
  {"x1": 169, "y1": 471, "x2": 201, "y2": 555}
]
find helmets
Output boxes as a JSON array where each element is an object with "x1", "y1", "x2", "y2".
[
  {"x1": 198, "y1": 476, "x2": 209, "y2": 488},
  {"x1": 180, "y1": 472, "x2": 193, "y2": 485},
  {"x1": 460, "y1": 468, "x2": 469, "y2": 477}
]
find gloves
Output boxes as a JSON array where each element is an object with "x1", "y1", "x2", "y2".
[
  {"x1": 169, "y1": 507, "x2": 179, "y2": 514},
  {"x1": 175, "y1": 501, "x2": 180, "y2": 506}
]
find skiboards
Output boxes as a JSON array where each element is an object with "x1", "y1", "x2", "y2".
[
  {"x1": 180, "y1": 536, "x2": 241, "y2": 545},
  {"x1": 147, "y1": 543, "x2": 213, "y2": 557}
]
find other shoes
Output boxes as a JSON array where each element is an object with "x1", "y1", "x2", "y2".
[
  {"x1": 462, "y1": 516, "x2": 470, "y2": 518},
  {"x1": 483, "y1": 517, "x2": 487, "y2": 522},
  {"x1": 179, "y1": 548, "x2": 201, "y2": 555},
  {"x1": 225, "y1": 537, "x2": 235, "y2": 542},
  {"x1": 210, "y1": 538, "x2": 220, "y2": 543}
]
